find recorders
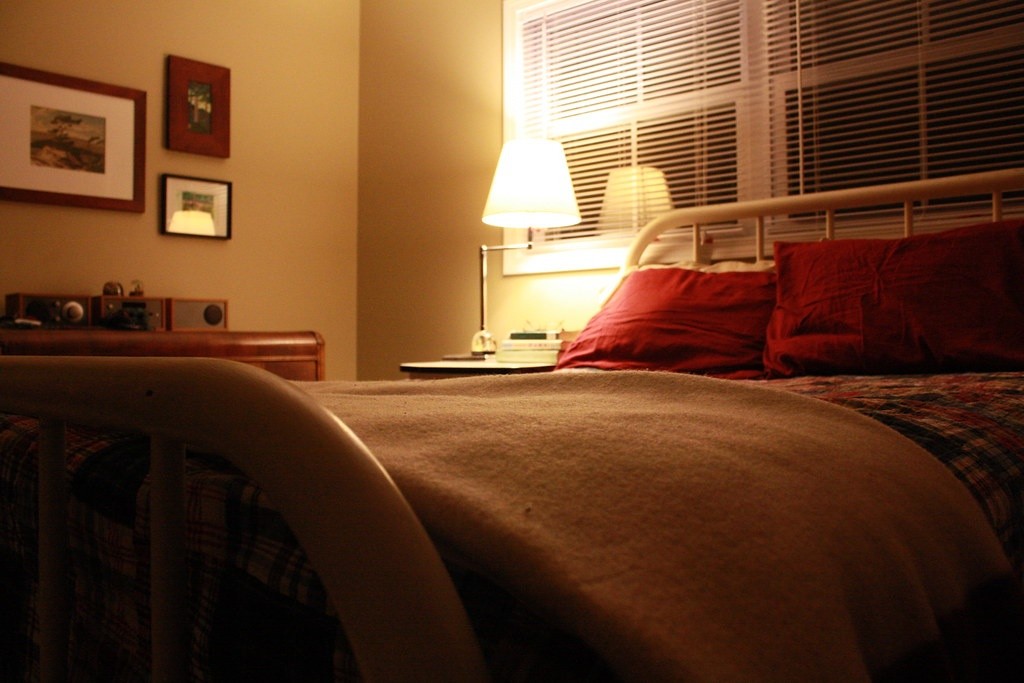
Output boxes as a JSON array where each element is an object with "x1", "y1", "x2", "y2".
[
  {"x1": 165, "y1": 297, "x2": 228, "y2": 332},
  {"x1": 5, "y1": 293, "x2": 91, "y2": 330},
  {"x1": 92, "y1": 295, "x2": 166, "y2": 332}
]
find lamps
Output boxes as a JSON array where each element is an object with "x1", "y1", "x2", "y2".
[{"x1": 444, "y1": 119, "x2": 583, "y2": 360}]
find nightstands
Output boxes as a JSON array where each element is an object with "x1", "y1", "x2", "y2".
[{"x1": 399, "y1": 359, "x2": 563, "y2": 381}]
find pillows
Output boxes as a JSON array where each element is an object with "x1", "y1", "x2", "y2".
[
  {"x1": 558, "y1": 262, "x2": 774, "y2": 377},
  {"x1": 765, "y1": 217, "x2": 1024, "y2": 378}
]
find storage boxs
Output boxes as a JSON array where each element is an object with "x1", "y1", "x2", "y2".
[
  {"x1": 4, "y1": 292, "x2": 93, "y2": 332},
  {"x1": 167, "y1": 297, "x2": 228, "y2": 332},
  {"x1": 93, "y1": 295, "x2": 166, "y2": 332}
]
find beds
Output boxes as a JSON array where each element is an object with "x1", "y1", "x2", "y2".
[{"x1": 0, "y1": 167, "x2": 1024, "y2": 683}]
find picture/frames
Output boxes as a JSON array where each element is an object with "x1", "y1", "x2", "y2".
[
  {"x1": 159, "y1": 172, "x2": 232, "y2": 241},
  {"x1": 0, "y1": 62, "x2": 145, "y2": 212},
  {"x1": 163, "y1": 53, "x2": 232, "y2": 159}
]
